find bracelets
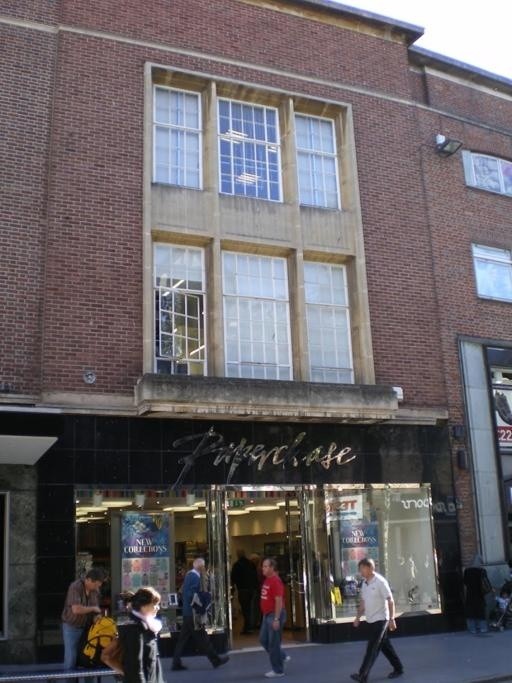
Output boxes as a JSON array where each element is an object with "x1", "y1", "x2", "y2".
[{"x1": 274, "y1": 618, "x2": 281, "y2": 621}]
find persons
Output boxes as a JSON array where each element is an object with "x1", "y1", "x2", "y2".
[
  {"x1": 351, "y1": 558, "x2": 403, "y2": 682},
  {"x1": 463, "y1": 556, "x2": 495, "y2": 633},
  {"x1": 258, "y1": 558, "x2": 291, "y2": 677},
  {"x1": 117, "y1": 588, "x2": 164, "y2": 682},
  {"x1": 491, "y1": 587, "x2": 511, "y2": 616},
  {"x1": 171, "y1": 558, "x2": 230, "y2": 671},
  {"x1": 231, "y1": 548, "x2": 264, "y2": 633},
  {"x1": 60, "y1": 568, "x2": 107, "y2": 671}
]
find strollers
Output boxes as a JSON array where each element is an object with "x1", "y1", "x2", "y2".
[{"x1": 489, "y1": 578, "x2": 512, "y2": 630}]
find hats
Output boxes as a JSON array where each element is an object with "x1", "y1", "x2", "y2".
[{"x1": 120, "y1": 590, "x2": 134, "y2": 599}]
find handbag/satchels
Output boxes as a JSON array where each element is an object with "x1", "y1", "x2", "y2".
[
  {"x1": 101, "y1": 635, "x2": 125, "y2": 675},
  {"x1": 80, "y1": 614, "x2": 118, "y2": 668}
]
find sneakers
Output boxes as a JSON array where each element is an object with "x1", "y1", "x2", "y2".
[
  {"x1": 471, "y1": 631, "x2": 494, "y2": 637},
  {"x1": 213, "y1": 655, "x2": 229, "y2": 668},
  {"x1": 282, "y1": 655, "x2": 291, "y2": 671},
  {"x1": 387, "y1": 668, "x2": 403, "y2": 679},
  {"x1": 170, "y1": 664, "x2": 187, "y2": 671},
  {"x1": 264, "y1": 670, "x2": 285, "y2": 678},
  {"x1": 351, "y1": 673, "x2": 367, "y2": 683}
]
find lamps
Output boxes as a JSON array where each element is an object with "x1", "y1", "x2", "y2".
[
  {"x1": 435, "y1": 134, "x2": 464, "y2": 155},
  {"x1": 185, "y1": 488, "x2": 196, "y2": 506},
  {"x1": 133, "y1": 490, "x2": 146, "y2": 509},
  {"x1": 92, "y1": 490, "x2": 104, "y2": 509}
]
